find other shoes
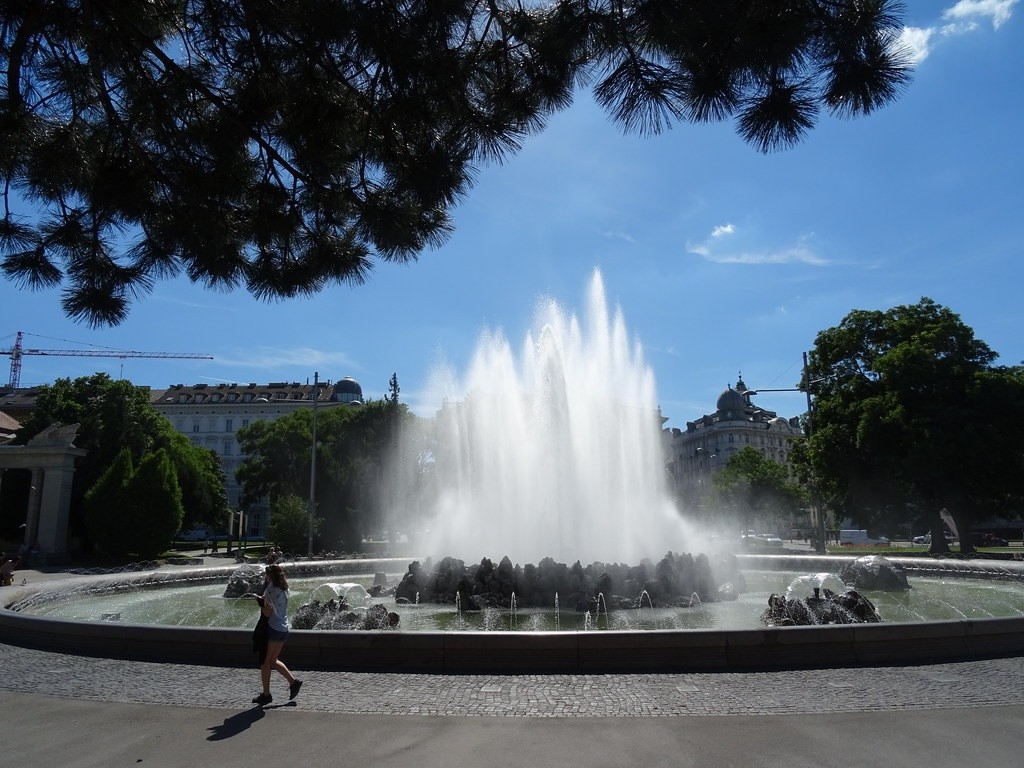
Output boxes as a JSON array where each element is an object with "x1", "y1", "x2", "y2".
[
  {"x1": 252, "y1": 692, "x2": 272, "y2": 703},
  {"x1": 289, "y1": 679, "x2": 303, "y2": 700}
]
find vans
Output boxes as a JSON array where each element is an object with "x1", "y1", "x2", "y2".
[{"x1": 840, "y1": 529, "x2": 890, "y2": 548}]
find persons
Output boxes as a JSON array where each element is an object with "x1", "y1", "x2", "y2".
[
  {"x1": 803, "y1": 531, "x2": 808, "y2": 542},
  {"x1": 0, "y1": 552, "x2": 9, "y2": 567},
  {"x1": 265, "y1": 547, "x2": 284, "y2": 564},
  {"x1": 204, "y1": 536, "x2": 218, "y2": 554},
  {"x1": 1012, "y1": 553, "x2": 1023, "y2": 561},
  {"x1": 322, "y1": 550, "x2": 340, "y2": 560},
  {"x1": 253, "y1": 564, "x2": 303, "y2": 703}
]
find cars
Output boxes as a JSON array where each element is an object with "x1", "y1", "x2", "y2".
[
  {"x1": 740, "y1": 529, "x2": 756, "y2": 543},
  {"x1": 969, "y1": 532, "x2": 1008, "y2": 548},
  {"x1": 913, "y1": 526, "x2": 960, "y2": 544},
  {"x1": 753, "y1": 533, "x2": 784, "y2": 547}
]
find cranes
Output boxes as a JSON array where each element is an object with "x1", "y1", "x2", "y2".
[{"x1": 0, "y1": 330, "x2": 214, "y2": 390}]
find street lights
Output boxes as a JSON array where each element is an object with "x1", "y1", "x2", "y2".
[
  {"x1": 255, "y1": 372, "x2": 362, "y2": 557},
  {"x1": 742, "y1": 352, "x2": 846, "y2": 556}
]
